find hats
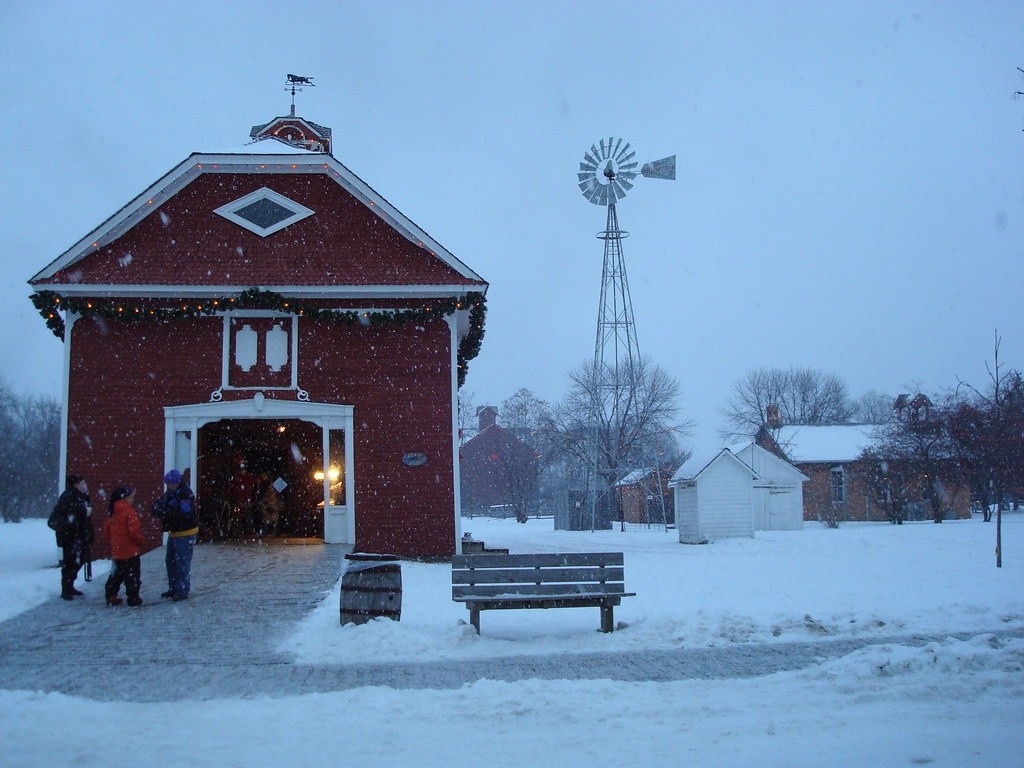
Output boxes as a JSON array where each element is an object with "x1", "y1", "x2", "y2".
[
  {"x1": 67, "y1": 475, "x2": 84, "y2": 486},
  {"x1": 163, "y1": 469, "x2": 181, "y2": 484},
  {"x1": 114, "y1": 486, "x2": 133, "y2": 500}
]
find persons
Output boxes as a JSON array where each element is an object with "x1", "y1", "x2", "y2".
[
  {"x1": 48, "y1": 474, "x2": 95, "y2": 601},
  {"x1": 153, "y1": 470, "x2": 201, "y2": 601},
  {"x1": 101, "y1": 481, "x2": 144, "y2": 606}
]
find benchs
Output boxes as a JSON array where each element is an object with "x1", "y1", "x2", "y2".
[{"x1": 452, "y1": 552, "x2": 637, "y2": 636}]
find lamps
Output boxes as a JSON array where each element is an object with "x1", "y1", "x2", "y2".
[{"x1": 276, "y1": 419, "x2": 285, "y2": 432}]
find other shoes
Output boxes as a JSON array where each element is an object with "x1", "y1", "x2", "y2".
[
  {"x1": 161, "y1": 586, "x2": 176, "y2": 597},
  {"x1": 173, "y1": 591, "x2": 188, "y2": 601},
  {"x1": 127, "y1": 597, "x2": 142, "y2": 606},
  {"x1": 106, "y1": 594, "x2": 122, "y2": 606}
]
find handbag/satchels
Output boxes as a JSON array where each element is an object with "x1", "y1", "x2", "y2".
[{"x1": 47, "y1": 492, "x2": 82, "y2": 537}]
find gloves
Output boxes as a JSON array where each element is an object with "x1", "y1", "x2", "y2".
[{"x1": 85, "y1": 507, "x2": 92, "y2": 516}]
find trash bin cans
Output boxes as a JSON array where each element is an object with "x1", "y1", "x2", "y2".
[{"x1": 339, "y1": 553, "x2": 402, "y2": 627}]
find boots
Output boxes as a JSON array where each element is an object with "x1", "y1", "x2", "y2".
[
  {"x1": 61, "y1": 578, "x2": 73, "y2": 601},
  {"x1": 72, "y1": 578, "x2": 83, "y2": 596}
]
